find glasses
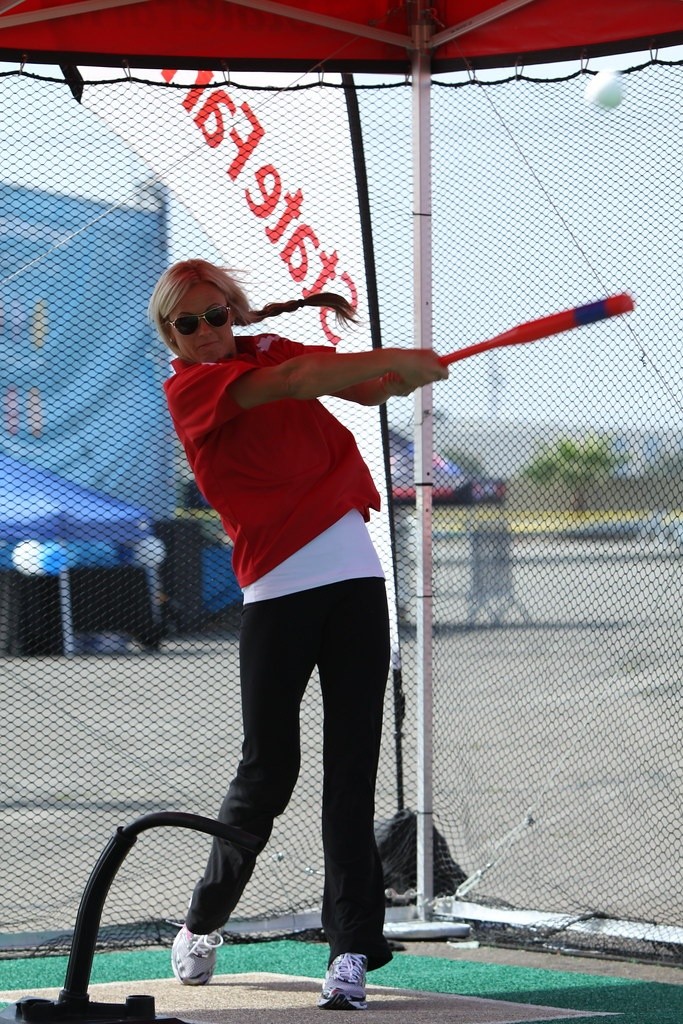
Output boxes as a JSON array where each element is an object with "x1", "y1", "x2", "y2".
[{"x1": 166, "y1": 303, "x2": 232, "y2": 335}]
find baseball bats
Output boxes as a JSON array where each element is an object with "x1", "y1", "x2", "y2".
[{"x1": 380, "y1": 291, "x2": 636, "y2": 390}]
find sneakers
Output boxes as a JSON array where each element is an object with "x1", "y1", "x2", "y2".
[
  {"x1": 171, "y1": 923, "x2": 224, "y2": 986},
  {"x1": 317, "y1": 953, "x2": 368, "y2": 1010}
]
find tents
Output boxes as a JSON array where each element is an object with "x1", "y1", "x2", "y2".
[{"x1": 0, "y1": 444, "x2": 153, "y2": 659}]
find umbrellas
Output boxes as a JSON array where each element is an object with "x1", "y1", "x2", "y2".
[
  {"x1": 178, "y1": 430, "x2": 465, "y2": 504},
  {"x1": 0, "y1": 0, "x2": 683, "y2": 921}
]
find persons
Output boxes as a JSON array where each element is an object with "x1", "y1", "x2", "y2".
[{"x1": 150, "y1": 259, "x2": 448, "y2": 1011}]
